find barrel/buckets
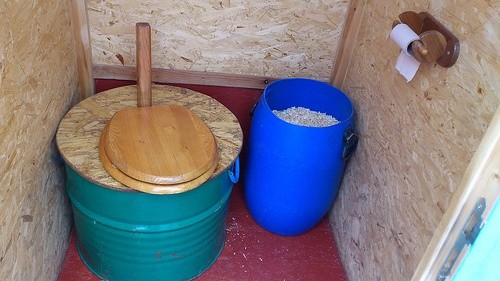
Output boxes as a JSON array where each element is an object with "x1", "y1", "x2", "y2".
[
  {"x1": 59, "y1": 128, "x2": 240, "y2": 281},
  {"x1": 245, "y1": 78, "x2": 356, "y2": 237}
]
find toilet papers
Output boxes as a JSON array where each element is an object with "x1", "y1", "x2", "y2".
[{"x1": 390, "y1": 24, "x2": 421, "y2": 82}]
